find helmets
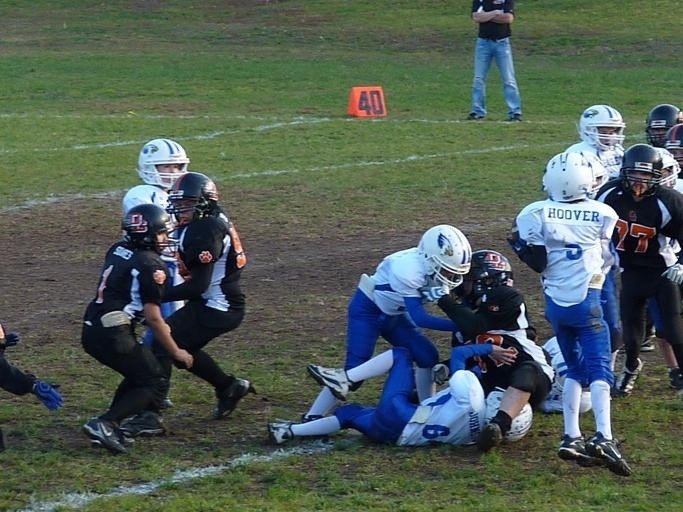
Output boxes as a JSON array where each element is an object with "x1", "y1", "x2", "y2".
[
  {"x1": 121, "y1": 204, "x2": 182, "y2": 259},
  {"x1": 136, "y1": 138, "x2": 191, "y2": 192},
  {"x1": 123, "y1": 184, "x2": 178, "y2": 230},
  {"x1": 620, "y1": 104, "x2": 683, "y2": 199},
  {"x1": 419, "y1": 225, "x2": 474, "y2": 292},
  {"x1": 458, "y1": 250, "x2": 515, "y2": 301},
  {"x1": 542, "y1": 152, "x2": 594, "y2": 203},
  {"x1": 484, "y1": 390, "x2": 534, "y2": 444},
  {"x1": 166, "y1": 172, "x2": 220, "y2": 228},
  {"x1": 579, "y1": 105, "x2": 627, "y2": 153}
]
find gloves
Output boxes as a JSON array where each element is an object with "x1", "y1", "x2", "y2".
[
  {"x1": 7, "y1": 333, "x2": 21, "y2": 347},
  {"x1": 661, "y1": 263, "x2": 683, "y2": 287},
  {"x1": 418, "y1": 274, "x2": 451, "y2": 306},
  {"x1": 34, "y1": 381, "x2": 66, "y2": 412}
]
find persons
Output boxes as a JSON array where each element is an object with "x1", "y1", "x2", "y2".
[
  {"x1": 81, "y1": 137, "x2": 250, "y2": 454},
  {"x1": 506, "y1": 102, "x2": 683, "y2": 477},
  {"x1": 0, "y1": 321, "x2": 64, "y2": 454},
  {"x1": 267, "y1": 224, "x2": 555, "y2": 463},
  {"x1": 466, "y1": 0, "x2": 522, "y2": 121}
]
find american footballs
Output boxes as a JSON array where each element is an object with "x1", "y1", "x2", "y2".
[{"x1": 512, "y1": 215, "x2": 517, "y2": 243}]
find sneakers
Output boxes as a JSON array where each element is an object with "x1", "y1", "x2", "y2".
[
  {"x1": 476, "y1": 423, "x2": 505, "y2": 454},
  {"x1": 307, "y1": 363, "x2": 355, "y2": 402},
  {"x1": 214, "y1": 376, "x2": 253, "y2": 421},
  {"x1": 467, "y1": 114, "x2": 484, "y2": 120},
  {"x1": 615, "y1": 325, "x2": 683, "y2": 395},
  {"x1": 557, "y1": 433, "x2": 605, "y2": 468},
  {"x1": 82, "y1": 395, "x2": 175, "y2": 455},
  {"x1": 511, "y1": 114, "x2": 523, "y2": 122},
  {"x1": 586, "y1": 432, "x2": 633, "y2": 478},
  {"x1": 301, "y1": 413, "x2": 336, "y2": 448},
  {"x1": 267, "y1": 421, "x2": 296, "y2": 447}
]
